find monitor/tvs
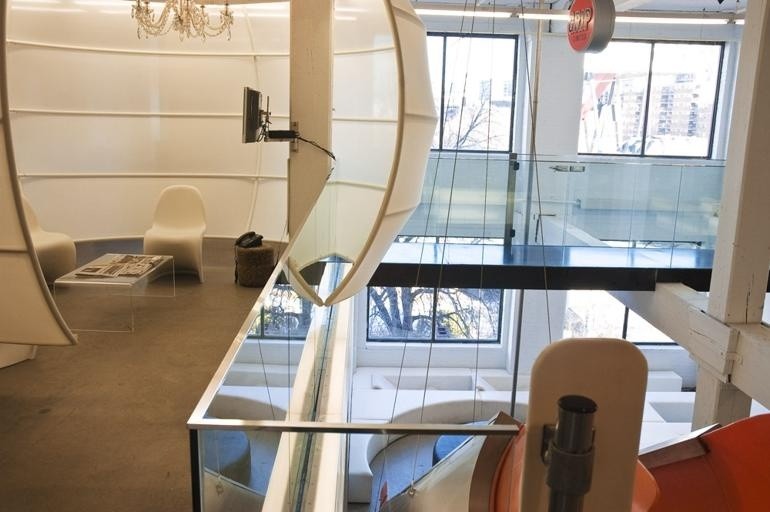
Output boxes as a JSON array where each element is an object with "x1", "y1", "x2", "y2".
[{"x1": 242, "y1": 86, "x2": 263, "y2": 145}]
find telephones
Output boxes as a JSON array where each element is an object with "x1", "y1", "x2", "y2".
[{"x1": 236, "y1": 231, "x2": 263, "y2": 248}]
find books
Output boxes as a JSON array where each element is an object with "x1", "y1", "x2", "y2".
[{"x1": 73, "y1": 252, "x2": 163, "y2": 278}]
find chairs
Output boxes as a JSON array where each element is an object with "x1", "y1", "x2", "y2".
[
  {"x1": 20, "y1": 193, "x2": 76, "y2": 286},
  {"x1": 142, "y1": 184, "x2": 206, "y2": 284}
]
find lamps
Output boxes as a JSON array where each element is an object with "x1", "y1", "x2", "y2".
[
  {"x1": 413, "y1": 2, "x2": 744, "y2": 25},
  {"x1": 130, "y1": 0, "x2": 235, "y2": 44}
]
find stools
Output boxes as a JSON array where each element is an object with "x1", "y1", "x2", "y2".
[
  {"x1": 432, "y1": 420, "x2": 493, "y2": 467},
  {"x1": 235, "y1": 243, "x2": 274, "y2": 288},
  {"x1": 197, "y1": 414, "x2": 250, "y2": 488}
]
